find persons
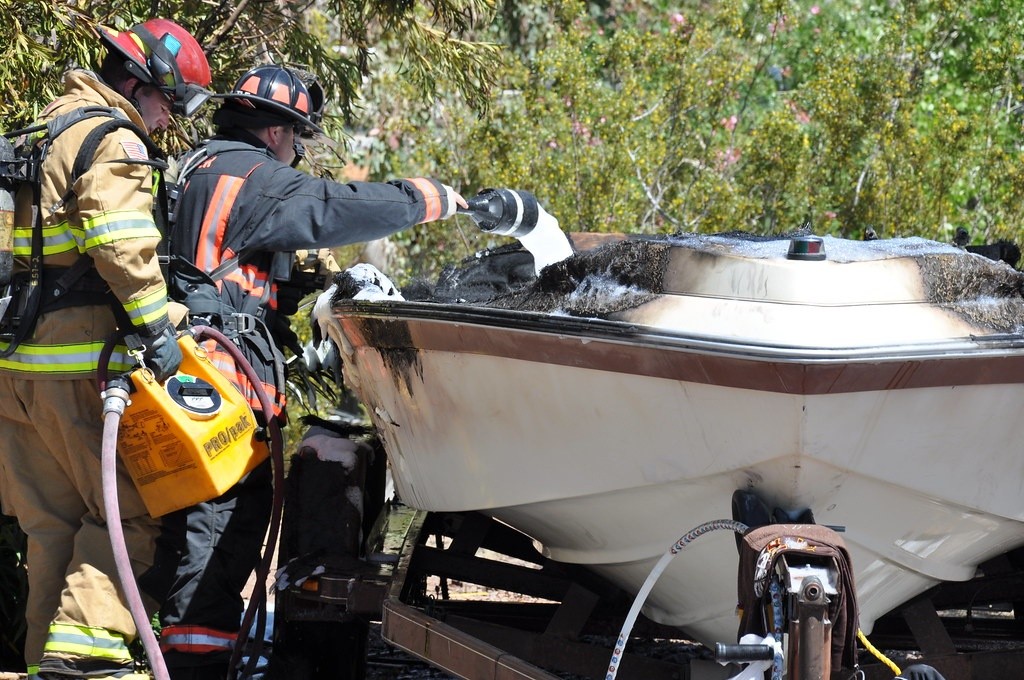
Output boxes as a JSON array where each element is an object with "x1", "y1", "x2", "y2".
[
  {"x1": 0, "y1": 19, "x2": 214, "y2": 680},
  {"x1": 157, "y1": 66, "x2": 468, "y2": 680}
]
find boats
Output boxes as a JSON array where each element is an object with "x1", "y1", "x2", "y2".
[{"x1": 275, "y1": 219, "x2": 1024, "y2": 652}]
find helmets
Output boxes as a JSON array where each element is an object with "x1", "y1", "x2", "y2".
[
  {"x1": 94, "y1": 20, "x2": 212, "y2": 108},
  {"x1": 211, "y1": 64, "x2": 326, "y2": 136}
]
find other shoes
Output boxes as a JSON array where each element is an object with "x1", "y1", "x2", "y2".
[{"x1": 37, "y1": 671, "x2": 150, "y2": 680}]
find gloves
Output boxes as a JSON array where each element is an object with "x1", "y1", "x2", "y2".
[{"x1": 131, "y1": 322, "x2": 183, "y2": 383}]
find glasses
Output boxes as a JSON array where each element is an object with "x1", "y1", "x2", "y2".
[{"x1": 173, "y1": 84, "x2": 215, "y2": 117}]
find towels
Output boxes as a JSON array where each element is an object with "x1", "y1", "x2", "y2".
[{"x1": 737, "y1": 524, "x2": 857, "y2": 671}]
